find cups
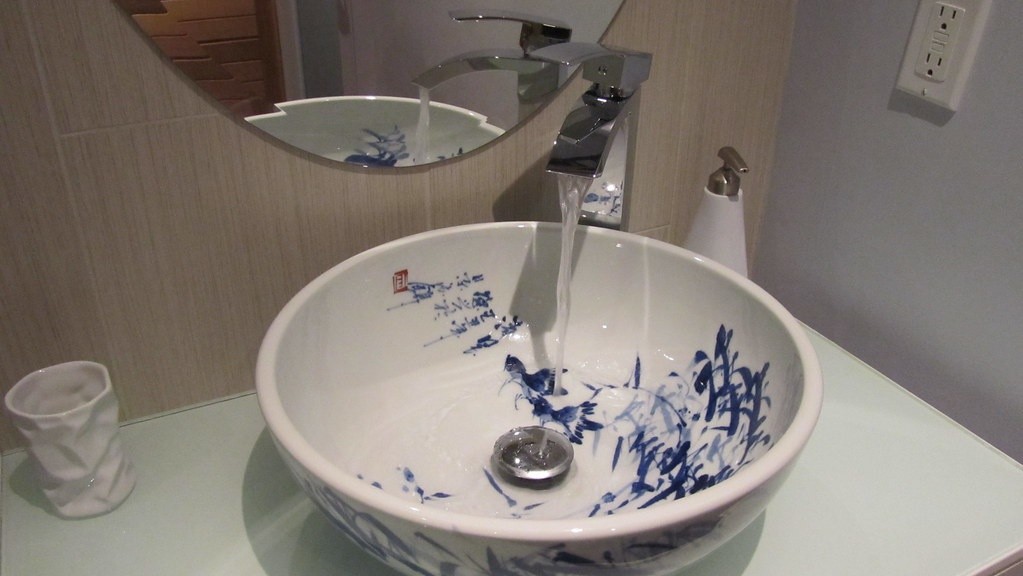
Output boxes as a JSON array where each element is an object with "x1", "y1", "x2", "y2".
[{"x1": 4, "y1": 360, "x2": 136, "y2": 519}]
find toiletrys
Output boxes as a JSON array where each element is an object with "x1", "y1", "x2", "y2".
[{"x1": 680, "y1": 145, "x2": 749, "y2": 280}]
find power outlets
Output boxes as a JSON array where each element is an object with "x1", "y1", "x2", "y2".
[{"x1": 889, "y1": 0, "x2": 997, "y2": 114}]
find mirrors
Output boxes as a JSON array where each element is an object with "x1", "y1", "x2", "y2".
[{"x1": 112, "y1": 0, "x2": 627, "y2": 172}]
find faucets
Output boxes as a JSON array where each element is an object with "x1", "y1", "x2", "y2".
[
  {"x1": 527, "y1": 41, "x2": 654, "y2": 233},
  {"x1": 410, "y1": 8, "x2": 575, "y2": 123}
]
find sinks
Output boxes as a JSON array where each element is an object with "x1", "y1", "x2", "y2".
[
  {"x1": 252, "y1": 220, "x2": 826, "y2": 576},
  {"x1": 243, "y1": 94, "x2": 506, "y2": 168}
]
